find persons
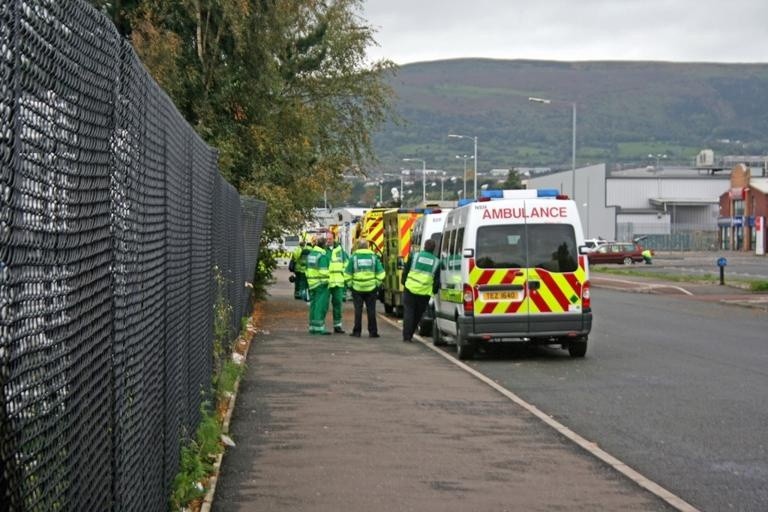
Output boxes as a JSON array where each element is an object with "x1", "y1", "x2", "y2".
[
  {"x1": 400, "y1": 238, "x2": 442, "y2": 343},
  {"x1": 342, "y1": 238, "x2": 387, "y2": 338},
  {"x1": 287, "y1": 231, "x2": 353, "y2": 336},
  {"x1": 641, "y1": 249, "x2": 655, "y2": 264}
]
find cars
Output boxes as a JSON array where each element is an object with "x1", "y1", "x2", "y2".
[{"x1": 583, "y1": 234, "x2": 648, "y2": 268}]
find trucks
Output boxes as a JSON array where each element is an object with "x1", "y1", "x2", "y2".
[
  {"x1": 353, "y1": 208, "x2": 392, "y2": 304},
  {"x1": 381, "y1": 208, "x2": 434, "y2": 319},
  {"x1": 408, "y1": 207, "x2": 456, "y2": 336},
  {"x1": 428, "y1": 188, "x2": 592, "y2": 361},
  {"x1": 331, "y1": 206, "x2": 375, "y2": 294}
]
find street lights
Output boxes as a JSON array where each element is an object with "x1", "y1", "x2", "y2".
[
  {"x1": 648, "y1": 153, "x2": 666, "y2": 175},
  {"x1": 528, "y1": 97, "x2": 576, "y2": 201},
  {"x1": 373, "y1": 133, "x2": 478, "y2": 208}
]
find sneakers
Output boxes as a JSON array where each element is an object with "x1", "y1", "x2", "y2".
[{"x1": 322, "y1": 327, "x2": 378, "y2": 337}]
